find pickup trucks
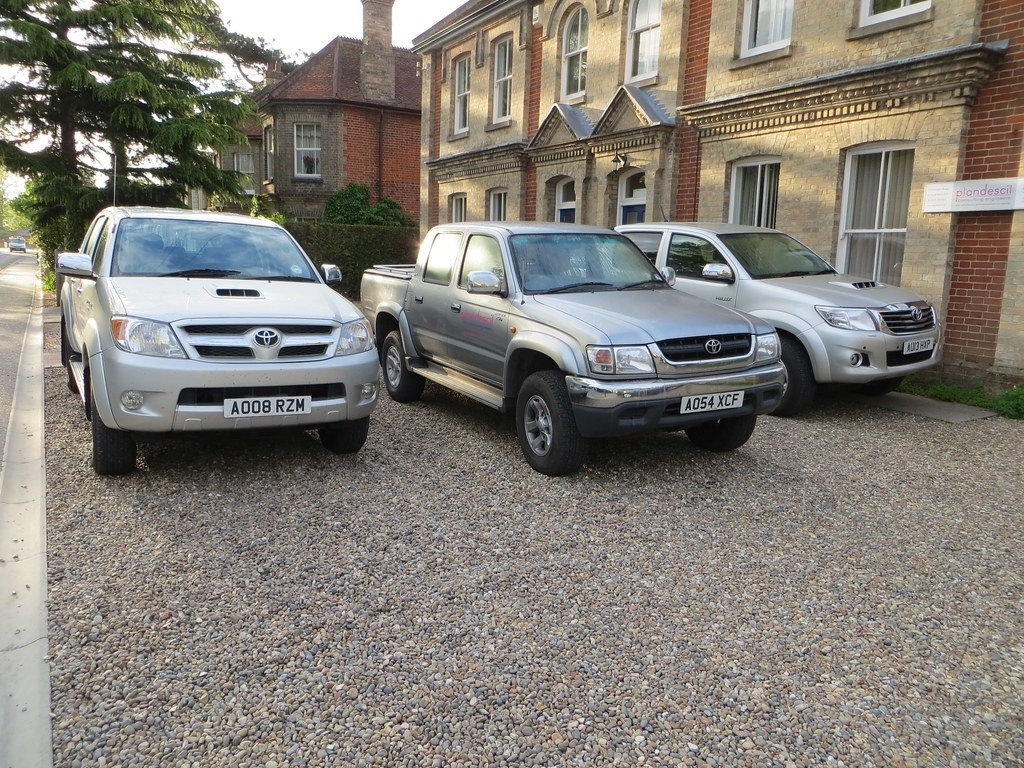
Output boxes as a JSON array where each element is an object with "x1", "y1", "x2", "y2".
[
  {"x1": 360, "y1": 222, "x2": 786, "y2": 476},
  {"x1": 565, "y1": 223, "x2": 945, "y2": 420}
]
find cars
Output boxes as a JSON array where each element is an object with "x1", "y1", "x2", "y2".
[{"x1": 54, "y1": 203, "x2": 380, "y2": 475}]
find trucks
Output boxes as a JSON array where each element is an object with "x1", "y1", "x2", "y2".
[{"x1": 9, "y1": 236, "x2": 26, "y2": 253}]
